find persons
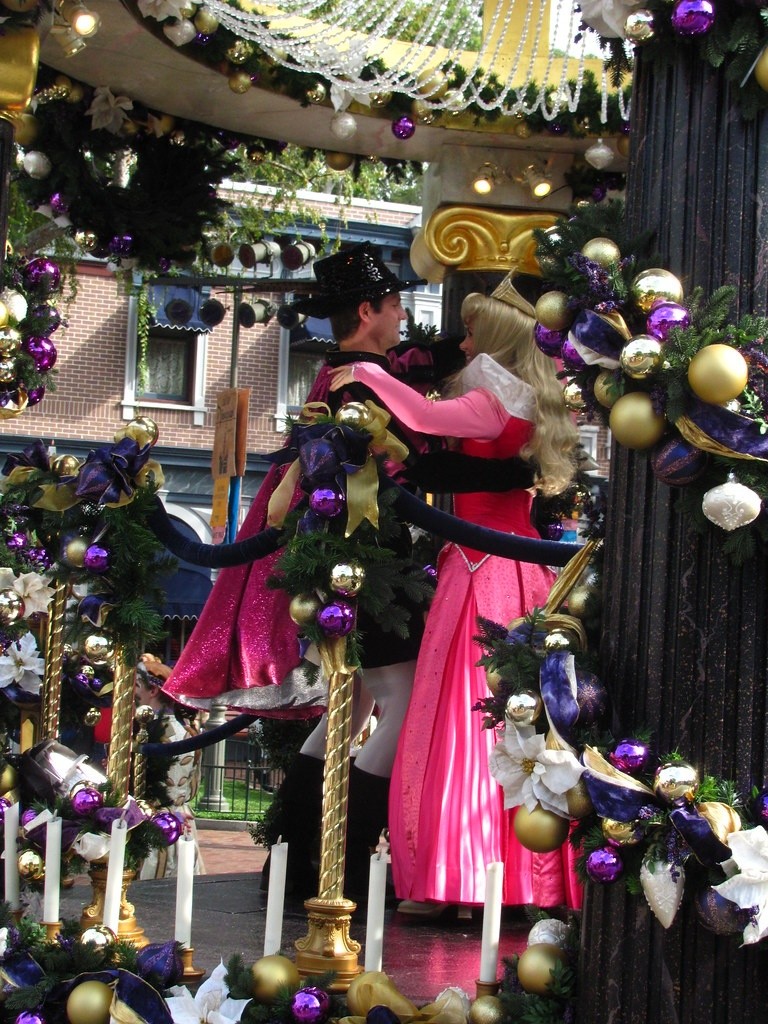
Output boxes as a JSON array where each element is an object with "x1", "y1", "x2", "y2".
[
  {"x1": 258, "y1": 244, "x2": 541, "y2": 922},
  {"x1": 326, "y1": 291, "x2": 583, "y2": 921},
  {"x1": 134, "y1": 661, "x2": 207, "y2": 880}
]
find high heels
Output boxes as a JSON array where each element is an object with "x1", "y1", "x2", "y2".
[{"x1": 397, "y1": 891, "x2": 471, "y2": 918}]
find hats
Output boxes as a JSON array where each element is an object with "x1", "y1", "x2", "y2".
[{"x1": 292, "y1": 240, "x2": 428, "y2": 319}]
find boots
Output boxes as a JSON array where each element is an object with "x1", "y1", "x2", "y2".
[
  {"x1": 257, "y1": 753, "x2": 336, "y2": 897},
  {"x1": 336, "y1": 756, "x2": 398, "y2": 906}
]
